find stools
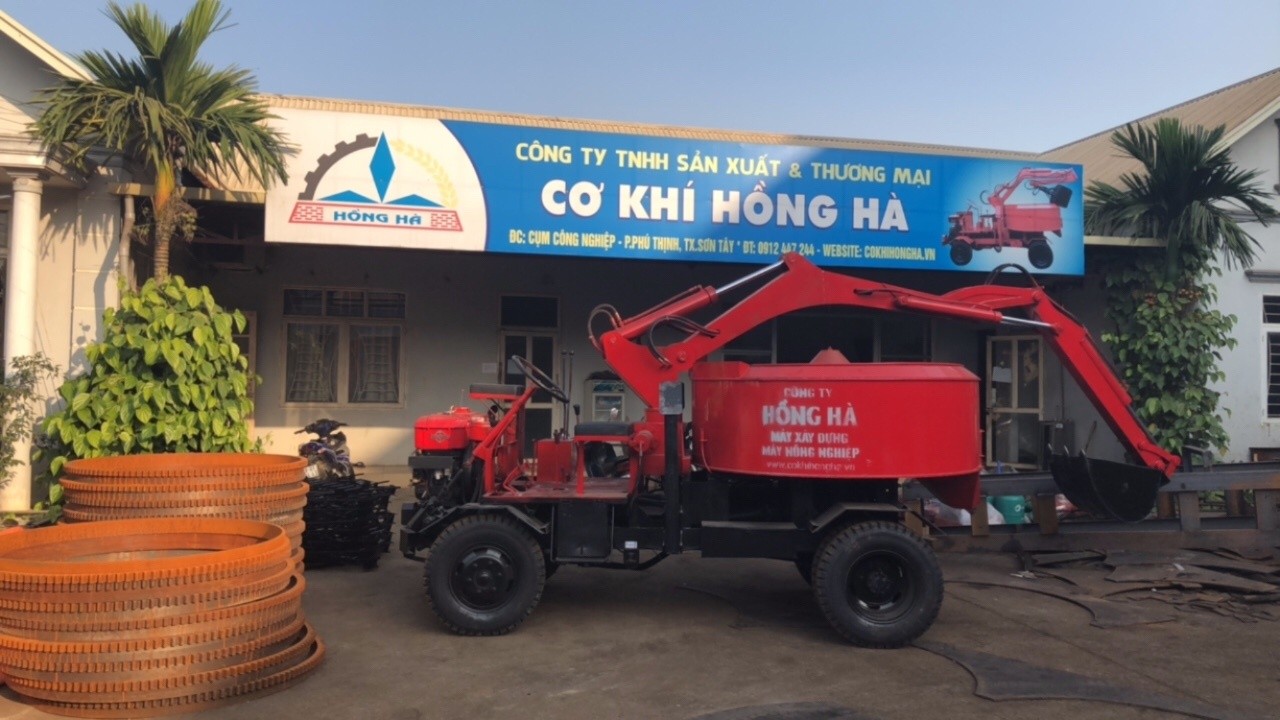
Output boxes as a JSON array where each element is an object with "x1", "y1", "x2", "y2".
[{"x1": 573, "y1": 422, "x2": 639, "y2": 493}]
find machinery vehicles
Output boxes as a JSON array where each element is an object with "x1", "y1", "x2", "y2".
[
  {"x1": 397, "y1": 250, "x2": 1188, "y2": 650},
  {"x1": 940, "y1": 167, "x2": 1078, "y2": 269}
]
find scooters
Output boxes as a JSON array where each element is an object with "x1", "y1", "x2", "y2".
[{"x1": 294, "y1": 417, "x2": 365, "y2": 480}]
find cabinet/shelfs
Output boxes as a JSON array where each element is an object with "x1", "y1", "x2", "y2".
[{"x1": 585, "y1": 379, "x2": 626, "y2": 422}]
